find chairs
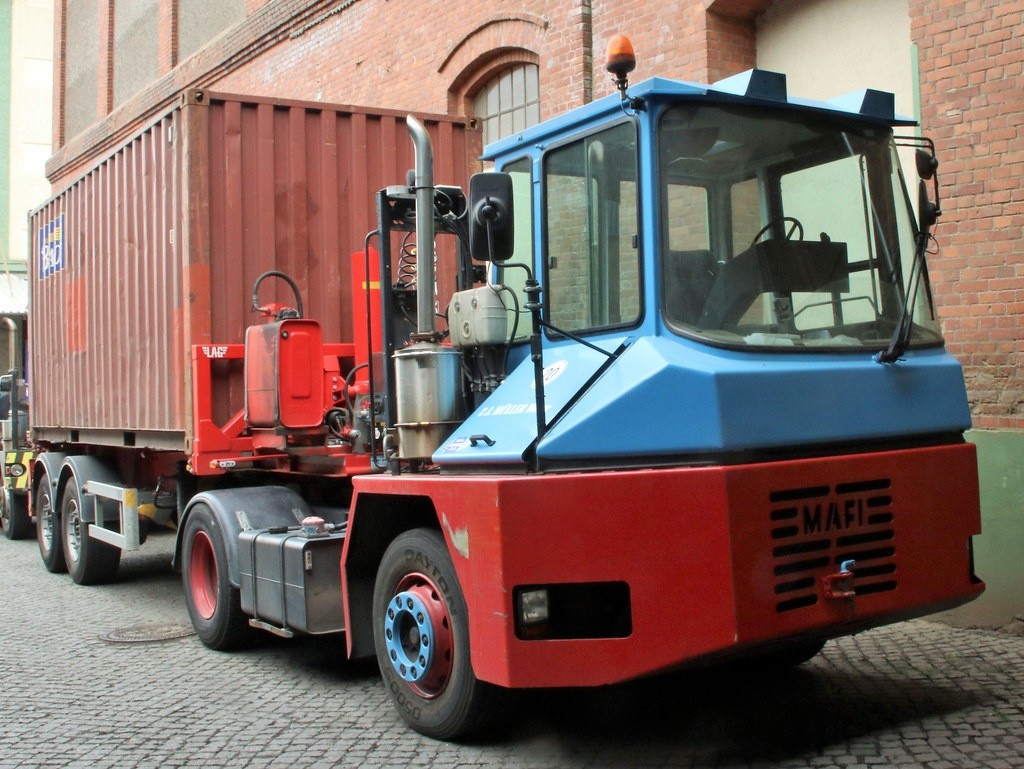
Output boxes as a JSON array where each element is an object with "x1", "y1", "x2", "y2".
[{"x1": 668, "y1": 248, "x2": 720, "y2": 324}]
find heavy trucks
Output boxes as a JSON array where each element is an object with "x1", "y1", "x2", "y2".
[{"x1": 0, "y1": 29, "x2": 991, "y2": 748}]
